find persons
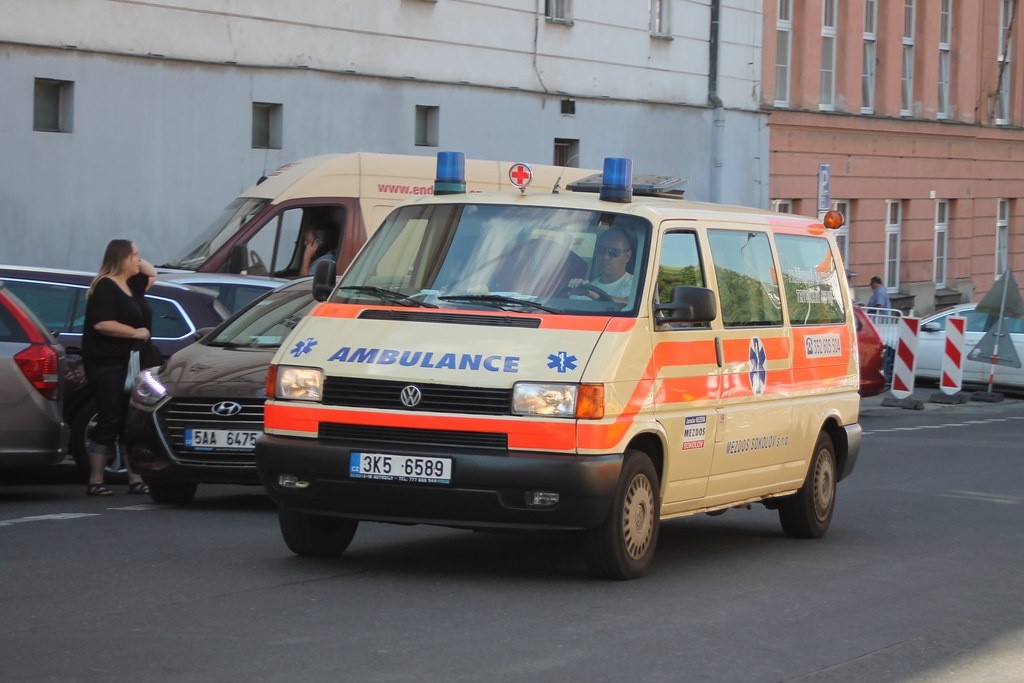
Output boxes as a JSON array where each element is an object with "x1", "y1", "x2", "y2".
[
  {"x1": 298, "y1": 219, "x2": 337, "y2": 277},
  {"x1": 567, "y1": 227, "x2": 633, "y2": 304},
  {"x1": 867, "y1": 276, "x2": 891, "y2": 315},
  {"x1": 79, "y1": 240, "x2": 157, "y2": 494}
]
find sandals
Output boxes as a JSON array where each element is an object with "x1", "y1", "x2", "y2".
[
  {"x1": 86, "y1": 483, "x2": 113, "y2": 497},
  {"x1": 128, "y1": 482, "x2": 149, "y2": 495}
]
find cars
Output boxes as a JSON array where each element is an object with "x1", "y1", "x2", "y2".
[
  {"x1": 124, "y1": 275, "x2": 344, "y2": 497},
  {"x1": 1, "y1": 264, "x2": 306, "y2": 477},
  {"x1": 918, "y1": 303, "x2": 1024, "y2": 395}
]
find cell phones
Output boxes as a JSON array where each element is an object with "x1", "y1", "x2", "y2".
[{"x1": 312, "y1": 238, "x2": 322, "y2": 247}]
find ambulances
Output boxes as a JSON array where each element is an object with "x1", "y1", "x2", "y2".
[
  {"x1": 151, "y1": 151, "x2": 602, "y2": 275},
  {"x1": 255, "y1": 151, "x2": 866, "y2": 584}
]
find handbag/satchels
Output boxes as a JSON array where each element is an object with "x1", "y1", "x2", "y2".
[{"x1": 123, "y1": 350, "x2": 141, "y2": 397}]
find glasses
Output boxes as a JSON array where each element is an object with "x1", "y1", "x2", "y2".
[{"x1": 596, "y1": 245, "x2": 630, "y2": 258}]
